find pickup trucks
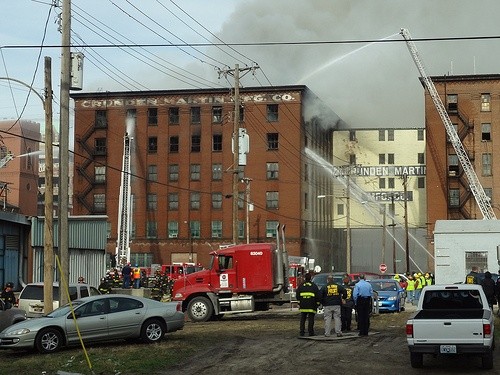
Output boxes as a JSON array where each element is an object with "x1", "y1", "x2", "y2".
[{"x1": 405, "y1": 284, "x2": 495, "y2": 370}]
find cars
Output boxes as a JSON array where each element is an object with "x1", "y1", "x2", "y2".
[
  {"x1": 0, "y1": 296, "x2": 27, "y2": 333},
  {"x1": 17, "y1": 282, "x2": 104, "y2": 320},
  {"x1": 310, "y1": 271, "x2": 407, "y2": 313},
  {"x1": 0, "y1": 294, "x2": 182, "y2": 354}
]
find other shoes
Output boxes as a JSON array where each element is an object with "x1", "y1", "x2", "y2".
[
  {"x1": 337, "y1": 333, "x2": 343, "y2": 336},
  {"x1": 364, "y1": 330, "x2": 368, "y2": 336},
  {"x1": 358, "y1": 330, "x2": 363, "y2": 336}
]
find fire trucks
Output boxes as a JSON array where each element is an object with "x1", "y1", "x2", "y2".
[{"x1": 103, "y1": 133, "x2": 204, "y2": 287}]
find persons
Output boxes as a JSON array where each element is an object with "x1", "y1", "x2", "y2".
[
  {"x1": 0, "y1": 282, "x2": 16, "y2": 310},
  {"x1": 465, "y1": 266, "x2": 500, "y2": 314},
  {"x1": 123, "y1": 132, "x2": 129, "y2": 155},
  {"x1": 78, "y1": 277, "x2": 86, "y2": 284},
  {"x1": 98, "y1": 252, "x2": 175, "y2": 302},
  {"x1": 297, "y1": 272, "x2": 435, "y2": 337}
]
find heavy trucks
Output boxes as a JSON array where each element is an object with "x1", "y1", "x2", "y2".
[{"x1": 170, "y1": 222, "x2": 380, "y2": 321}]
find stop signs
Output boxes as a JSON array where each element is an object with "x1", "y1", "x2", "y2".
[{"x1": 379, "y1": 264, "x2": 387, "y2": 272}]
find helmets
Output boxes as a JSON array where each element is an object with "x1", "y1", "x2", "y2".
[
  {"x1": 135, "y1": 264, "x2": 139, "y2": 266},
  {"x1": 78, "y1": 277, "x2": 84, "y2": 281},
  {"x1": 101, "y1": 277, "x2": 105, "y2": 280},
  {"x1": 6, "y1": 282, "x2": 14, "y2": 288},
  {"x1": 128, "y1": 262, "x2": 131, "y2": 265},
  {"x1": 409, "y1": 277, "x2": 413, "y2": 279},
  {"x1": 415, "y1": 275, "x2": 419, "y2": 278},
  {"x1": 343, "y1": 278, "x2": 349, "y2": 283},
  {"x1": 418, "y1": 273, "x2": 421, "y2": 276}
]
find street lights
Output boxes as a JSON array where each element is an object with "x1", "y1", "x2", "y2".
[
  {"x1": 317, "y1": 193, "x2": 351, "y2": 276},
  {"x1": 224, "y1": 176, "x2": 252, "y2": 244},
  {"x1": 382, "y1": 222, "x2": 397, "y2": 263}
]
